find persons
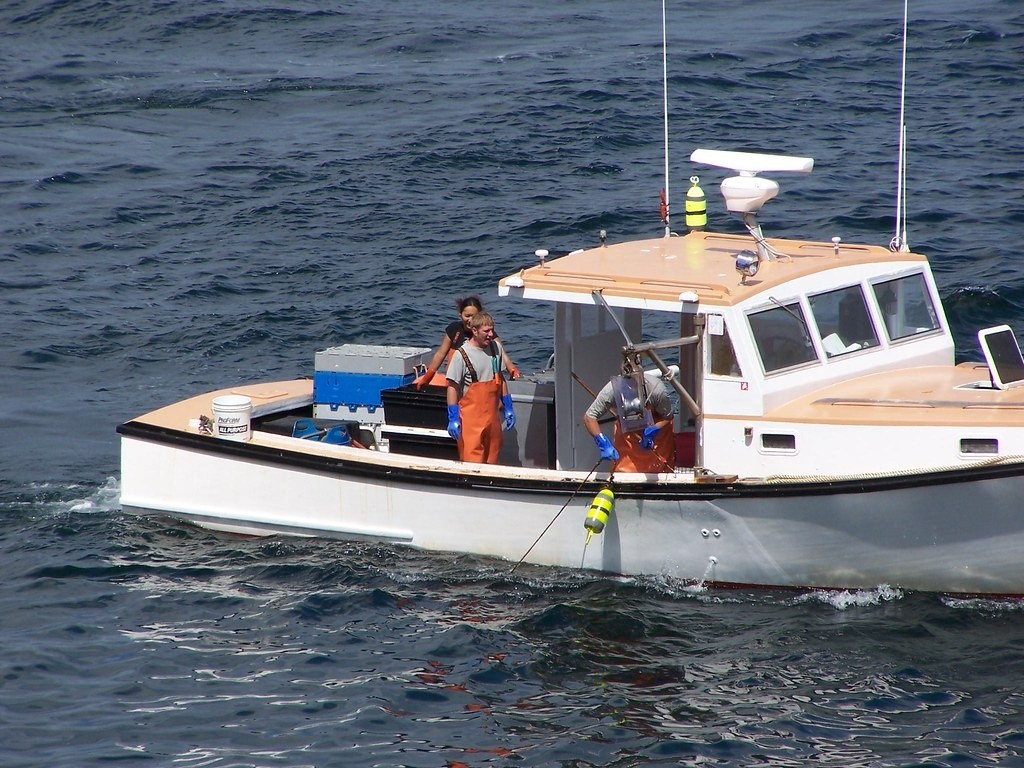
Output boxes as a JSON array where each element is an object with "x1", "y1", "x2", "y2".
[
  {"x1": 417, "y1": 297, "x2": 520, "y2": 391},
  {"x1": 445, "y1": 311, "x2": 516, "y2": 464},
  {"x1": 585, "y1": 373, "x2": 676, "y2": 472}
]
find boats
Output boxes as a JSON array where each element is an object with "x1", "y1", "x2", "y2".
[{"x1": 113, "y1": 2, "x2": 1024, "y2": 608}]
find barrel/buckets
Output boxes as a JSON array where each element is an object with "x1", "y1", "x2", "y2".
[
  {"x1": 321, "y1": 424, "x2": 351, "y2": 447},
  {"x1": 211, "y1": 394, "x2": 252, "y2": 442},
  {"x1": 292, "y1": 417, "x2": 322, "y2": 441}
]
